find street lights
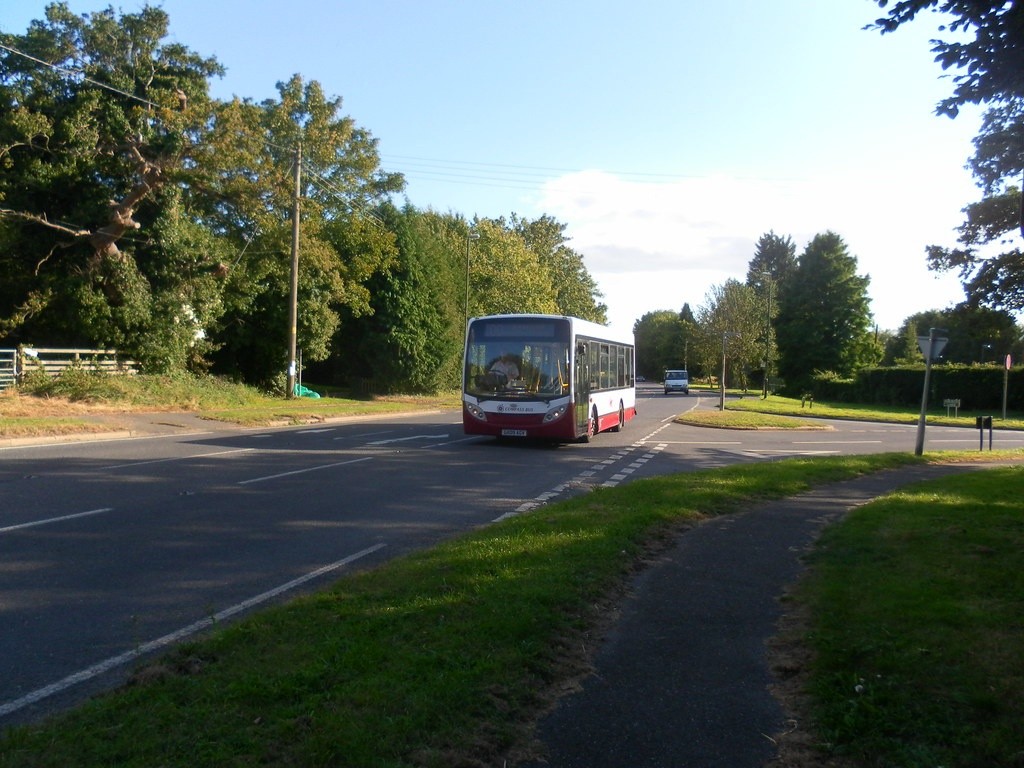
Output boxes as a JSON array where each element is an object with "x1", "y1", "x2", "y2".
[
  {"x1": 463, "y1": 233, "x2": 481, "y2": 347},
  {"x1": 761, "y1": 271, "x2": 773, "y2": 398}
]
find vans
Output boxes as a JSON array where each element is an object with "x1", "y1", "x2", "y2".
[{"x1": 663, "y1": 369, "x2": 690, "y2": 395}]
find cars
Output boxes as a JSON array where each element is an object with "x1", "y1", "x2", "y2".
[{"x1": 636, "y1": 375, "x2": 645, "y2": 382}]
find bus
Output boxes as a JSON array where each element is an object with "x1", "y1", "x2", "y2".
[{"x1": 459, "y1": 313, "x2": 638, "y2": 443}]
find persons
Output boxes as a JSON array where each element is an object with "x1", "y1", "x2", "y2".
[{"x1": 489, "y1": 349, "x2": 519, "y2": 381}]
